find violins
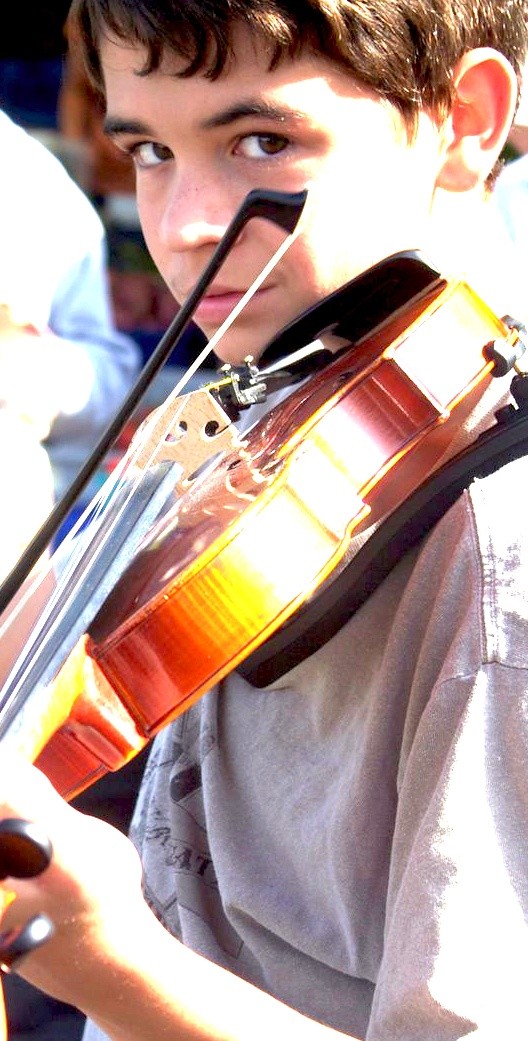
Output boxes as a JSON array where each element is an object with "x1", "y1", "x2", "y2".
[{"x1": 0, "y1": 276, "x2": 523, "y2": 818}]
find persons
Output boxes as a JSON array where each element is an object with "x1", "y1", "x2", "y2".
[{"x1": 1, "y1": 0, "x2": 528, "y2": 1041}]
